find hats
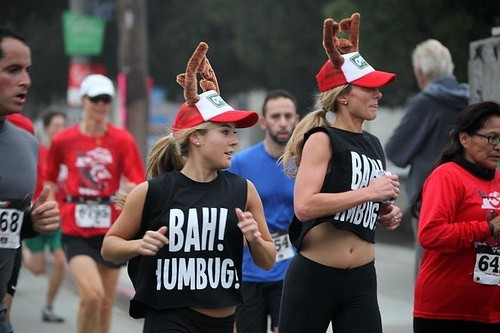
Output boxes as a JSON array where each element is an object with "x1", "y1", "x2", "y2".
[
  {"x1": 171, "y1": 42, "x2": 259, "y2": 137},
  {"x1": 79, "y1": 73, "x2": 115, "y2": 103},
  {"x1": 315, "y1": 12, "x2": 397, "y2": 93}
]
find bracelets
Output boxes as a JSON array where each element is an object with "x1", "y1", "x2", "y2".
[{"x1": 488, "y1": 222, "x2": 494, "y2": 236}]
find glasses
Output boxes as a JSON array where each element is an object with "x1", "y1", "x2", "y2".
[
  {"x1": 85, "y1": 94, "x2": 113, "y2": 106},
  {"x1": 468, "y1": 130, "x2": 500, "y2": 146}
]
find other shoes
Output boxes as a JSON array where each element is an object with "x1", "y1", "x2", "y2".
[{"x1": 41, "y1": 306, "x2": 64, "y2": 323}]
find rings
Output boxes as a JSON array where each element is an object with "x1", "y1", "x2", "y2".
[
  {"x1": 393, "y1": 216, "x2": 399, "y2": 222},
  {"x1": 395, "y1": 186, "x2": 399, "y2": 193}
]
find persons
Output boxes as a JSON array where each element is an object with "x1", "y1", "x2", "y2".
[
  {"x1": 0, "y1": 27, "x2": 500, "y2": 333},
  {"x1": 276, "y1": 51, "x2": 403, "y2": 333},
  {"x1": 100, "y1": 90, "x2": 276, "y2": 333}
]
